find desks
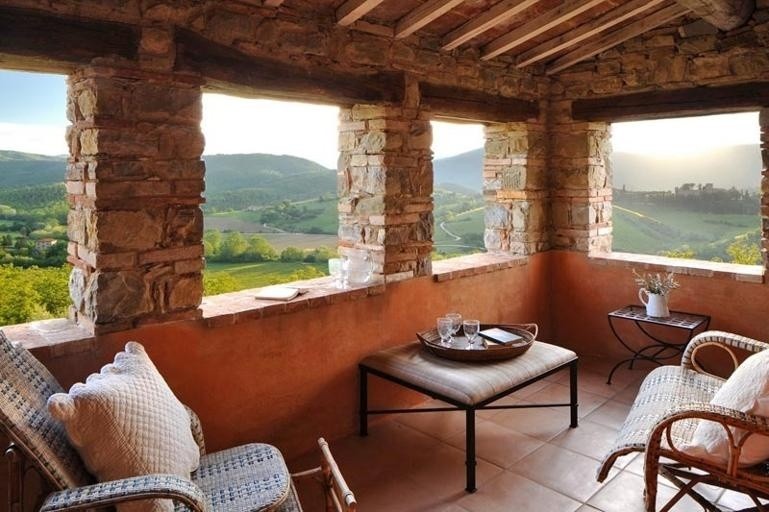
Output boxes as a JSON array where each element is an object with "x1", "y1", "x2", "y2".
[
  {"x1": 605, "y1": 303, "x2": 711, "y2": 384},
  {"x1": 353, "y1": 334, "x2": 582, "y2": 496}
]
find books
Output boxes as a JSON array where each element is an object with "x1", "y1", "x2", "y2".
[
  {"x1": 254, "y1": 284, "x2": 312, "y2": 301},
  {"x1": 477, "y1": 327, "x2": 522, "y2": 345},
  {"x1": 482, "y1": 339, "x2": 527, "y2": 348}
]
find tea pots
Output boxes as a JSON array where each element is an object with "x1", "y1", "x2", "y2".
[
  {"x1": 637, "y1": 286, "x2": 672, "y2": 318},
  {"x1": 338, "y1": 245, "x2": 376, "y2": 288}
]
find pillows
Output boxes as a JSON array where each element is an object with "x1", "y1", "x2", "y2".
[
  {"x1": 44, "y1": 340, "x2": 204, "y2": 512},
  {"x1": 677, "y1": 344, "x2": 769, "y2": 472}
]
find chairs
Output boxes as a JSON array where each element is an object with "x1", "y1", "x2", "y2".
[
  {"x1": 592, "y1": 325, "x2": 769, "y2": 512},
  {"x1": 0, "y1": 326, "x2": 364, "y2": 512}
]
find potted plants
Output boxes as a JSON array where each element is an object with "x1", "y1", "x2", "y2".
[{"x1": 630, "y1": 265, "x2": 682, "y2": 320}]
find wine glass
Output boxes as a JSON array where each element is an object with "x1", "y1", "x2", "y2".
[
  {"x1": 436, "y1": 317, "x2": 452, "y2": 349},
  {"x1": 444, "y1": 313, "x2": 461, "y2": 344},
  {"x1": 461, "y1": 318, "x2": 479, "y2": 351}
]
